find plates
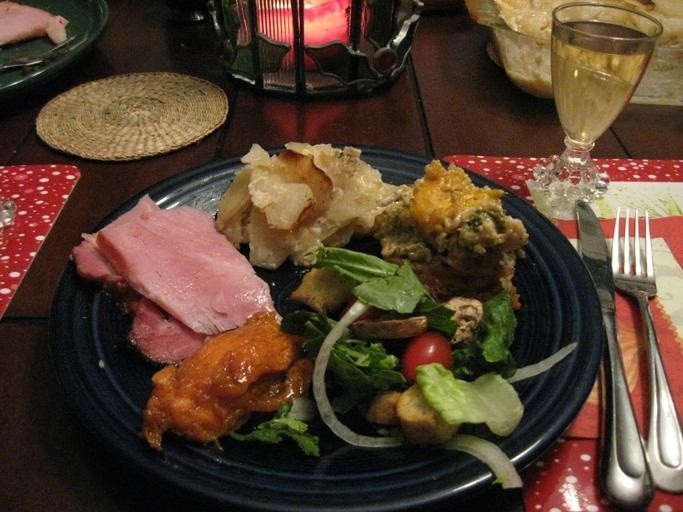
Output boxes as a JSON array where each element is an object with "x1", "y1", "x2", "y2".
[
  {"x1": 1, "y1": 2, "x2": 108, "y2": 89},
  {"x1": 47, "y1": 144, "x2": 602, "y2": 512}
]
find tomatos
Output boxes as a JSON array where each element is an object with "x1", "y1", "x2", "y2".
[{"x1": 403, "y1": 331, "x2": 452, "y2": 382}]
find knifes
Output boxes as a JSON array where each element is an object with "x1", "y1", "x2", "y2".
[{"x1": 576, "y1": 198, "x2": 654, "y2": 512}]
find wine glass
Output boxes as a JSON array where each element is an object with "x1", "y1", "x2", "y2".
[{"x1": 537, "y1": 1, "x2": 663, "y2": 218}]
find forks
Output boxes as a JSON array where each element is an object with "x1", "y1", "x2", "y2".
[{"x1": 610, "y1": 205, "x2": 683, "y2": 493}]
